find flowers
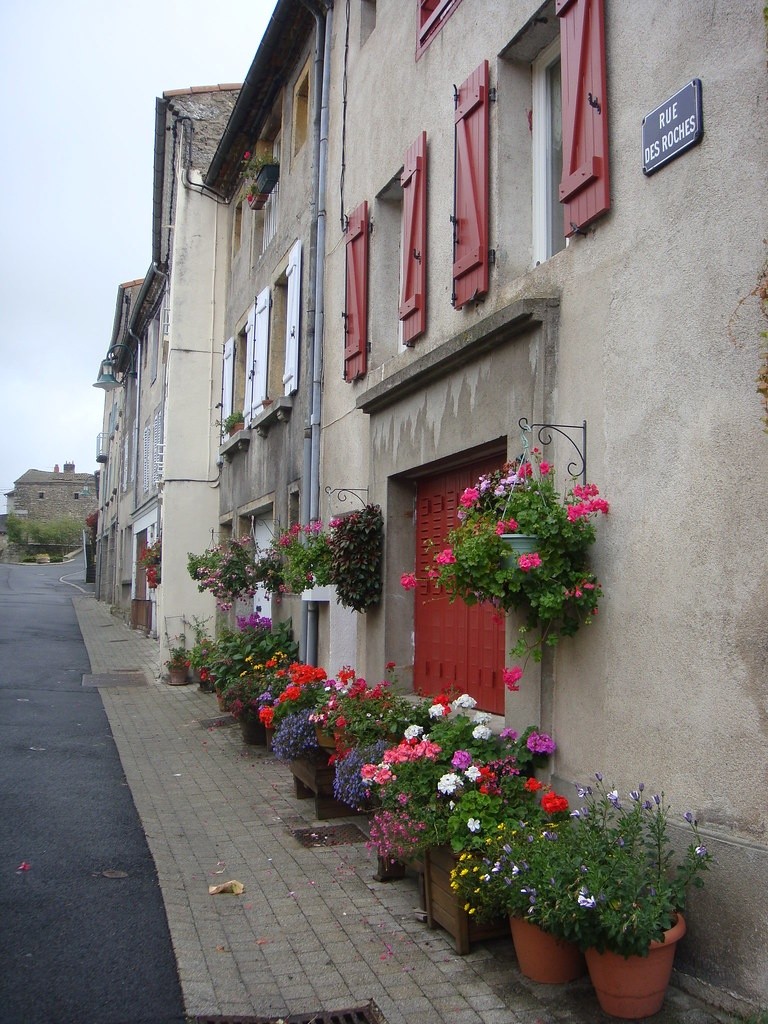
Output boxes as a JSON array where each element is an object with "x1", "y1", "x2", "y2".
[
  {"x1": 163, "y1": 448, "x2": 718, "y2": 962},
  {"x1": 85, "y1": 512, "x2": 97, "y2": 537},
  {"x1": 236, "y1": 151, "x2": 280, "y2": 211},
  {"x1": 135, "y1": 546, "x2": 162, "y2": 587}
]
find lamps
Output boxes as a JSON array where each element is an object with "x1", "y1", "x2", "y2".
[{"x1": 93, "y1": 345, "x2": 138, "y2": 392}]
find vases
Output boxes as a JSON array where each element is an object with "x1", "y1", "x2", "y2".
[
  {"x1": 491, "y1": 534, "x2": 535, "y2": 580},
  {"x1": 253, "y1": 166, "x2": 278, "y2": 192},
  {"x1": 168, "y1": 665, "x2": 191, "y2": 687},
  {"x1": 509, "y1": 908, "x2": 585, "y2": 984},
  {"x1": 424, "y1": 840, "x2": 510, "y2": 956},
  {"x1": 240, "y1": 713, "x2": 265, "y2": 746},
  {"x1": 265, "y1": 725, "x2": 279, "y2": 752},
  {"x1": 574, "y1": 916, "x2": 685, "y2": 1018},
  {"x1": 198, "y1": 681, "x2": 211, "y2": 691},
  {"x1": 246, "y1": 192, "x2": 267, "y2": 210},
  {"x1": 375, "y1": 853, "x2": 425, "y2": 914},
  {"x1": 315, "y1": 724, "x2": 336, "y2": 749}
]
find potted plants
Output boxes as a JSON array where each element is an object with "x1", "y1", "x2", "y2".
[
  {"x1": 211, "y1": 413, "x2": 244, "y2": 437},
  {"x1": 261, "y1": 396, "x2": 272, "y2": 409}
]
